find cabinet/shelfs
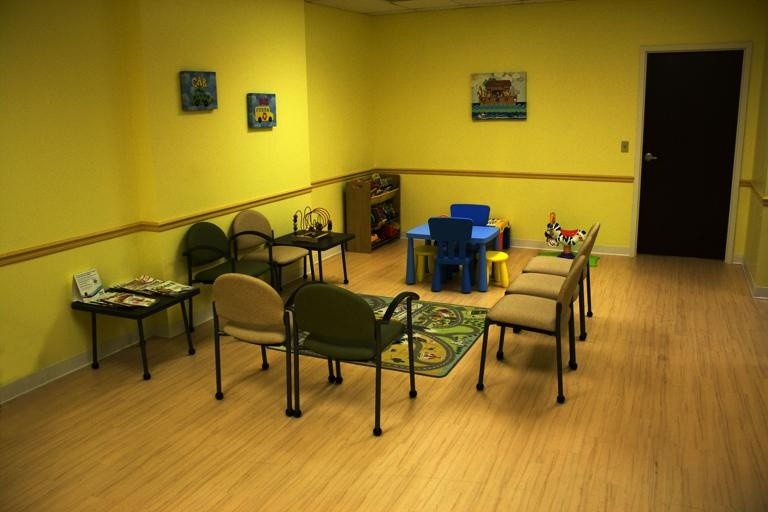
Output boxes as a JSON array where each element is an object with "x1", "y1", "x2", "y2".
[{"x1": 345, "y1": 173, "x2": 401, "y2": 254}]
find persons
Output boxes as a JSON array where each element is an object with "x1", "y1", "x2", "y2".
[{"x1": 73, "y1": 269, "x2": 106, "y2": 304}]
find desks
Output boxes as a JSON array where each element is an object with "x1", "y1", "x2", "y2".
[{"x1": 71, "y1": 279, "x2": 200, "y2": 380}]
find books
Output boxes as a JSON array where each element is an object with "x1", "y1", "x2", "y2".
[{"x1": 90, "y1": 274, "x2": 197, "y2": 310}]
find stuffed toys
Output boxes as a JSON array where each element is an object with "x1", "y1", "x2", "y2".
[{"x1": 353, "y1": 173, "x2": 401, "y2": 247}]
